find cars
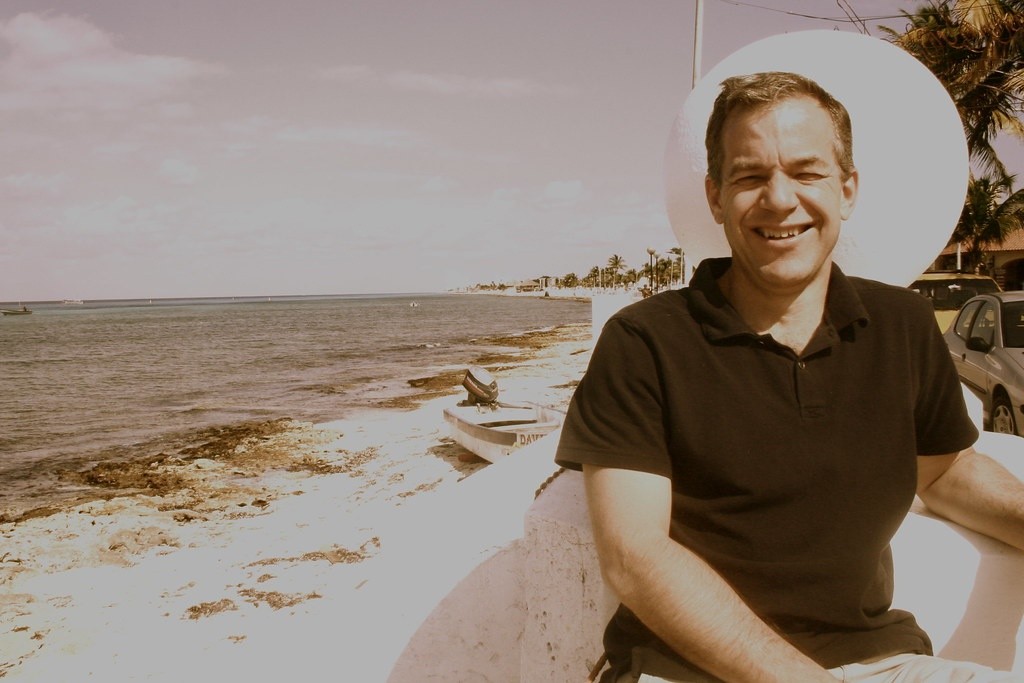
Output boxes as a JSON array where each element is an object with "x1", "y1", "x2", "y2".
[
  {"x1": 906, "y1": 269, "x2": 1004, "y2": 336},
  {"x1": 941, "y1": 290, "x2": 1024, "y2": 438}
]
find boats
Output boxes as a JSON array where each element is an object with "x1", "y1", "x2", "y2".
[
  {"x1": 441, "y1": 363, "x2": 567, "y2": 465},
  {"x1": 0, "y1": 305, "x2": 33, "y2": 315}
]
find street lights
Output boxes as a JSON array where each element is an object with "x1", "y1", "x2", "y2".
[
  {"x1": 646, "y1": 246, "x2": 656, "y2": 295},
  {"x1": 653, "y1": 251, "x2": 660, "y2": 294}
]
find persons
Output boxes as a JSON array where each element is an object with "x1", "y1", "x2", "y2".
[{"x1": 555, "y1": 69, "x2": 1024, "y2": 683}]
